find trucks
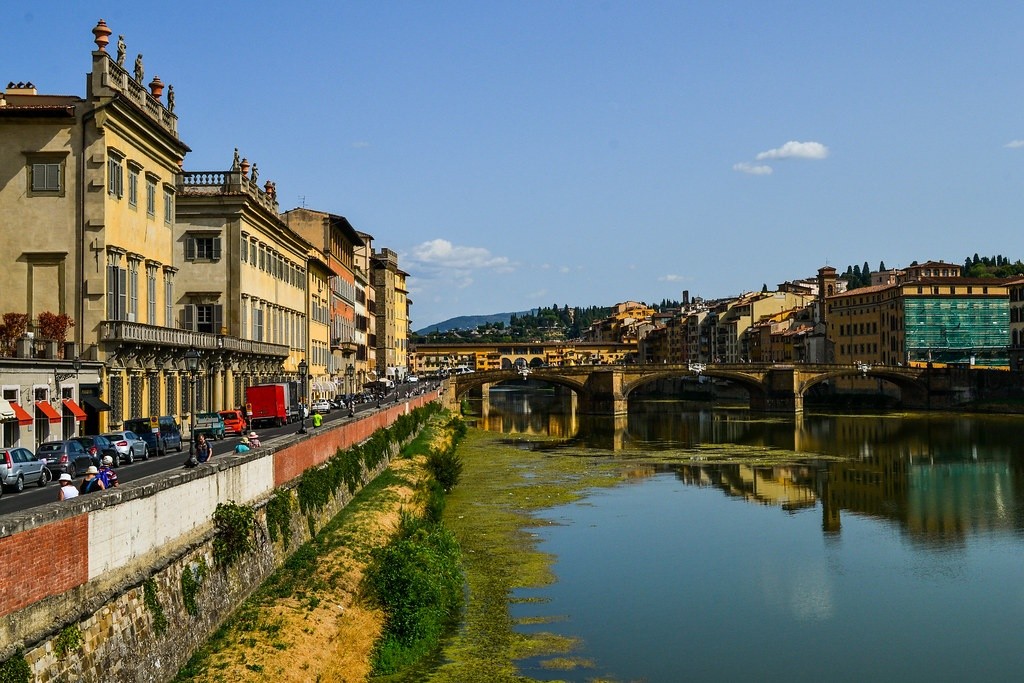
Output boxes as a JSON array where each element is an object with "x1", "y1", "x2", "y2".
[
  {"x1": 258, "y1": 381, "x2": 300, "y2": 423},
  {"x1": 245, "y1": 385, "x2": 288, "y2": 430}
]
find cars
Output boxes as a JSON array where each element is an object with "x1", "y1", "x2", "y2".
[
  {"x1": 68, "y1": 435, "x2": 119, "y2": 469},
  {"x1": 99, "y1": 430, "x2": 150, "y2": 465},
  {"x1": 378, "y1": 375, "x2": 425, "y2": 390},
  {"x1": 311, "y1": 398, "x2": 330, "y2": 413},
  {"x1": 217, "y1": 410, "x2": 248, "y2": 437},
  {"x1": 35, "y1": 440, "x2": 96, "y2": 481}
]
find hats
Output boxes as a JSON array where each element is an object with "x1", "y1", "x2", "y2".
[
  {"x1": 59, "y1": 473, "x2": 72, "y2": 481},
  {"x1": 240, "y1": 437, "x2": 250, "y2": 443},
  {"x1": 314, "y1": 409, "x2": 320, "y2": 413},
  {"x1": 248, "y1": 432, "x2": 259, "y2": 438},
  {"x1": 83, "y1": 466, "x2": 100, "y2": 474},
  {"x1": 102, "y1": 456, "x2": 114, "y2": 465}
]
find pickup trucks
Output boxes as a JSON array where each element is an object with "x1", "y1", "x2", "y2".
[{"x1": 191, "y1": 412, "x2": 225, "y2": 441}]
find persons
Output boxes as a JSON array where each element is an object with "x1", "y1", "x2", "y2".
[
  {"x1": 59, "y1": 474, "x2": 78, "y2": 500},
  {"x1": 247, "y1": 432, "x2": 260, "y2": 449},
  {"x1": 96, "y1": 456, "x2": 118, "y2": 488},
  {"x1": 79, "y1": 466, "x2": 105, "y2": 494},
  {"x1": 312, "y1": 410, "x2": 322, "y2": 429},
  {"x1": 233, "y1": 438, "x2": 249, "y2": 453},
  {"x1": 195, "y1": 433, "x2": 212, "y2": 462}
]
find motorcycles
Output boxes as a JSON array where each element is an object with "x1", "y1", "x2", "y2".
[{"x1": 328, "y1": 390, "x2": 386, "y2": 409}]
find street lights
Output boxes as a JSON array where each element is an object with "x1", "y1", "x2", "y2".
[
  {"x1": 297, "y1": 358, "x2": 309, "y2": 434},
  {"x1": 183, "y1": 346, "x2": 200, "y2": 468},
  {"x1": 375, "y1": 364, "x2": 437, "y2": 409},
  {"x1": 347, "y1": 363, "x2": 355, "y2": 418}
]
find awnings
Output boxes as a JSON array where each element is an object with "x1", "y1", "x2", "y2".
[
  {"x1": 83, "y1": 398, "x2": 111, "y2": 411},
  {"x1": 10, "y1": 403, "x2": 33, "y2": 425},
  {"x1": 36, "y1": 402, "x2": 61, "y2": 423},
  {"x1": 63, "y1": 400, "x2": 86, "y2": 420},
  {"x1": 0, "y1": 397, "x2": 15, "y2": 420}
]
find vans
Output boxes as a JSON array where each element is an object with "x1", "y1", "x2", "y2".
[
  {"x1": 121, "y1": 415, "x2": 183, "y2": 456},
  {"x1": 447, "y1": 367, "x2": 475, "y2": 376}
]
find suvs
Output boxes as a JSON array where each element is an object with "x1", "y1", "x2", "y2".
[{"x1": 1, "y1": 446, "x2": 47, "y2": 493}]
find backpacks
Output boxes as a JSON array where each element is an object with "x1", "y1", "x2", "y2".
[{"x1": 96, "y1": 469, "x2": 111, "y2": 488}]
